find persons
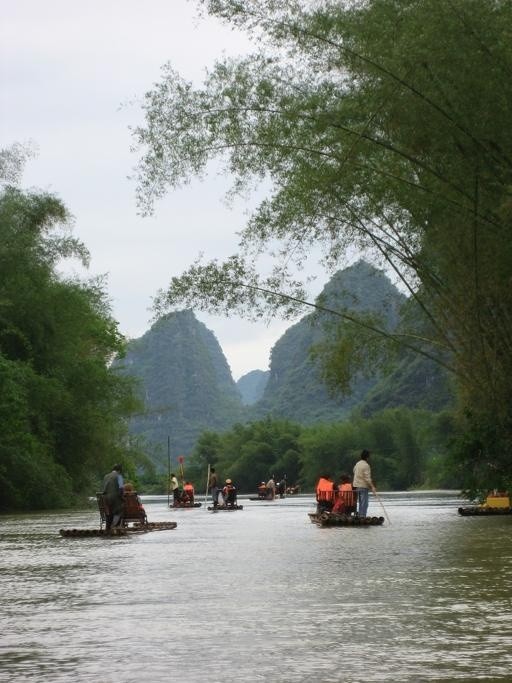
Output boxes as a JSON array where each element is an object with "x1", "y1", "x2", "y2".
[
  {"x1": 259, "y1": 473, "x2": 300, "y2": 501},
  {"x1": 102, "y1": 464, "x2": 146, "y2": 531},
  {"x1": 208, "y1": 468, "x2": 237, "y2": 507},
  {"x1": 168, "y1": 473, "x2": 194, "y2": 507},
  {"x1": 314, "y1": 472, "x2": 357, "y2": 517},
  {"x1": 487, "y1": 486, "x2": 509, "y2": 498},
  {"x1": 352, "y1": 449, "x2": 377, "y2": 519}
]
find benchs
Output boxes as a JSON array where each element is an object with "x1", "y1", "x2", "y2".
[
  {"x1": 96, "y1": 489, "x2": 148, "y2": 530},
  {"x1": 173, "y1": 488, "x2": 193, "y2": 504},
  {"x1": 257, "y1": 487, "x2": 274, "y2": 497},
  {"x1": 317, "y1": 490, "x2": 359, "y2": 518},
  {"x1": 214, "y1": 489, "x2": 238, "y2": 507}
]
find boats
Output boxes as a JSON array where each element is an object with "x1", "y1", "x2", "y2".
[
  {"x1": 60, "y1": 491, "x2": 177, "y2": 537},
  {"x1": 307, "y1": 489, "x2": 384, "y2": 527},
  {"x1": 170, "y1": 487, "x2": 278, "y2": 509},
  {"x1": 458, "y1": 488, "x2": 512, "y2": 516}
]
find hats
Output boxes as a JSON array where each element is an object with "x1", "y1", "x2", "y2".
[{"x1": 225, "y1": 479, "x2": 231, "y2": 484}]
